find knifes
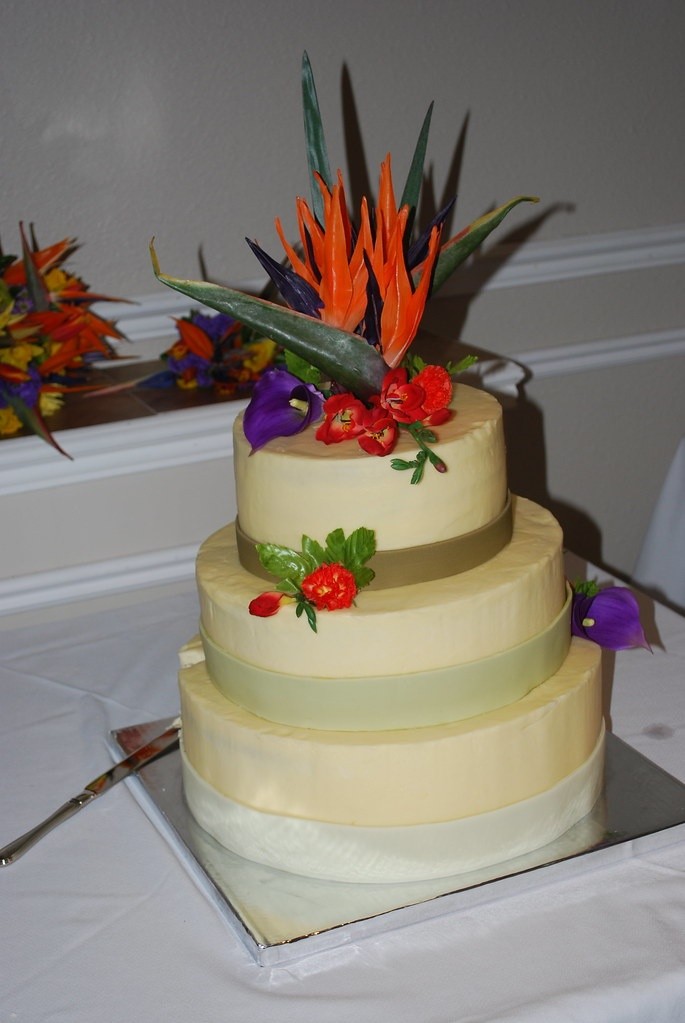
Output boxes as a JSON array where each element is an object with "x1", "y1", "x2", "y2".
[{"x1": 0, "y1": 726, "x2": 179, "y2": 867}]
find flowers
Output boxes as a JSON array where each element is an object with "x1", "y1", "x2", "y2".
[
  {"x1": 249, "y1": 527, "x2": 378, "y2": 638},
  {"x1": 568, "y1": 574, "x2": 655, "y2": 656},
  {"x1": 0, "y1": 216, "x2": 143, "y2": 461},
  {"x1": 142, "y1": 244, "x2": 320, "y2": 405},
  {"x1": 240, "y1": 50, "x2": 546, "y2": 485}
]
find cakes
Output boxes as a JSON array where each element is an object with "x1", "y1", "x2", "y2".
[{"x1": 176, "y1": 380, "x2": 607, "y2": 885}]
